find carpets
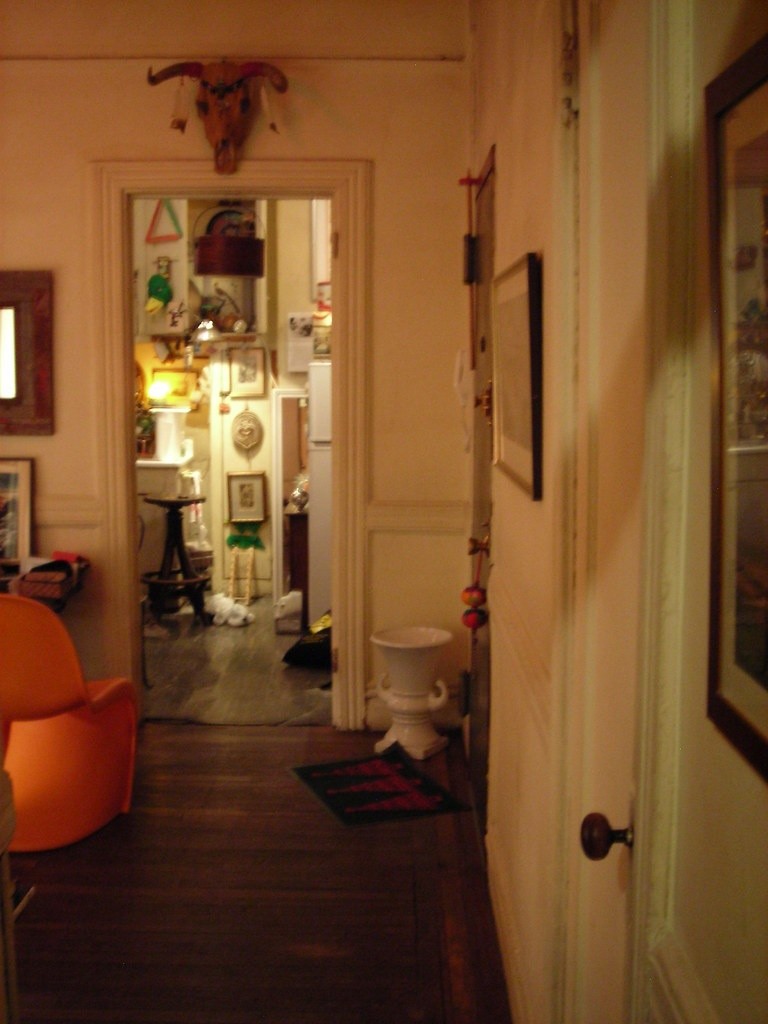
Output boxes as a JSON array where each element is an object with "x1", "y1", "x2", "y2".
[
  {"x1": 144, "y1": 595, "x2": 337, "y2": 725},
  {"x1": 292, "y1": 739, "x2": 472, "y2": 829}
]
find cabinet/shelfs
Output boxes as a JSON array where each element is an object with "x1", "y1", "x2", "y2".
[{"x1": 131, "y1": 197, "x2": 274, "y2": 344}]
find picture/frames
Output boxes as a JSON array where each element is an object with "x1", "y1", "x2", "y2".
[
  {"x1": 227, "y1": 472, "x2": 266, "y2": 523},
  {"x1": 0, "y1": 455, "x2": 36, "y2": 564},
  {"x1": 230, "y1": 345, "x2": 268, "y2": 398},
  {"x1": 491, "y1": 250, "x2": 543, "y2": 499},
  {"x1": 706, "y1": 37, "x2": 768, "y2": 785}
]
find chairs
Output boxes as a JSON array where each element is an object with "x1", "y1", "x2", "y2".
[{"x1": 0, "y1": 592, "x2": 141, "y2": 853}]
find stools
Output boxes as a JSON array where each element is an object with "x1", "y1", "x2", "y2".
[{"x1": 143, "y1": 493, "x2": 214, "y2": 626}]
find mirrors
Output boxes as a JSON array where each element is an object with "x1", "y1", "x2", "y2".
[{"x1": 0, "y1": 269, "x2": 54, "y2": 436}]
